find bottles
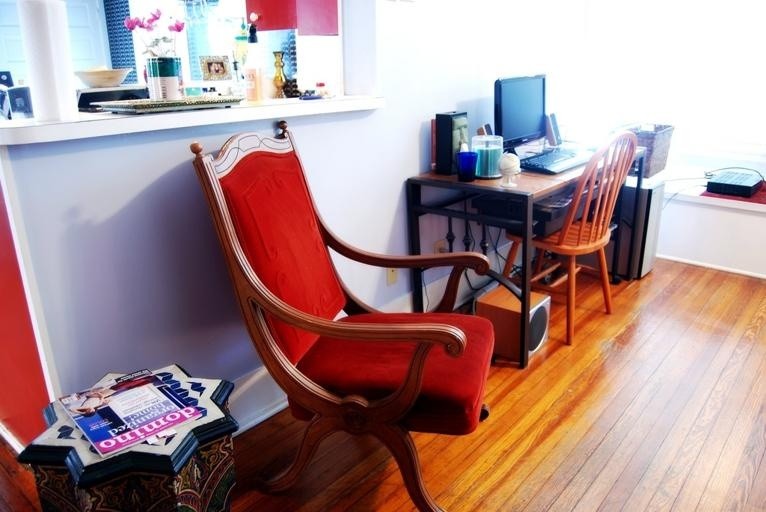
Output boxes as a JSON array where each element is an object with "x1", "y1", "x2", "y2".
[{"x1": 241, "y1": 23, "x2": 267, "y2": 104}]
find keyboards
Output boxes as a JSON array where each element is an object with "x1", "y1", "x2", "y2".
[{"x1": 519, "y1": 148, "x2": 593, "y2": 174}]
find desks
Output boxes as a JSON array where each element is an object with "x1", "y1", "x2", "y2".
[
  {"x1": 14, "y1": 363, "x2": 239, "y2": 510},
  {"x1": 405, "y1": 137, "x2": 647, "y2": 371}
]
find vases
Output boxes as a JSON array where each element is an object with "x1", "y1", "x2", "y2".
[{"x1": 144, "y1": 57, "x2": 185, "y2": 100}]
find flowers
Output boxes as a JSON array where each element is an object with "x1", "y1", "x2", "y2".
[{"x1": 120, "y1": 5, "x2": 186, "y2": 55}]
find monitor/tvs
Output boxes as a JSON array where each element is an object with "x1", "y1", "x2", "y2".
[{"x1": 493, "y1": 71, "x2": 548, "y2": 156}]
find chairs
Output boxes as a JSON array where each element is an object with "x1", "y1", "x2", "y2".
[
  {"x1": 191, "y1": 122, "x2": 495, "y2": 512},
  {"x1": 500, "y1": 130, "x2": 638, "y2": 345}
]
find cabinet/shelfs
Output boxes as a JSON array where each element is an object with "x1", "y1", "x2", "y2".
[{"x1": 244, "y1": 1, "x2": 339, "y2": 35}]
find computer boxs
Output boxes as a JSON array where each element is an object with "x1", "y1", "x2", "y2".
[{"x1": 550, "y1": 177, "x2": 665, "y2": 278}]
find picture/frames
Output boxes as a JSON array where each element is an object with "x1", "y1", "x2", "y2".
[{"x1": 198, "y1": 55, "x2": 234, "y2": 82}]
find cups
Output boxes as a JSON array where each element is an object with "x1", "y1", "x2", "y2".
[{"x1": 455, "y1": 152, "x2": 478, "y2": 182}]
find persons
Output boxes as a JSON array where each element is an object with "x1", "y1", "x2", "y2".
[
  {"x1": 214, "y1": 64, "x2": 219, "y2": 73},
  {"x1": 69, "y1": 390, "x2": 109, "y2": 418},
  {"x1": 209, "y1": 63, "x2": 215, "y2": 73},
  {"x1": 218, "y1": 63, "x2": 224, "y2": 73}
]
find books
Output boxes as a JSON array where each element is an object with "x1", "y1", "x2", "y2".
[
  {"x1": 57, "y1": 367, "x2": 203, "y2": 459},
  {"x1": 431, "y1": 117, "x2": 438, "y2": 172}
]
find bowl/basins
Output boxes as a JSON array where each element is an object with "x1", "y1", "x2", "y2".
[{"x1": 72, "y1": 67, "x2": 132, "y2": 88}]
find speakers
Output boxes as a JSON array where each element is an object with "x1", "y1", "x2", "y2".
[
  {"x1": 475, "y1": 287, "x2": 552, "y2": 363},
  {"x1": 547, "y1": 112, "x2": 565, "y2": 146},
  {"x1": 478, "y1": 124, "x2": 493, "y2": 137}
]
